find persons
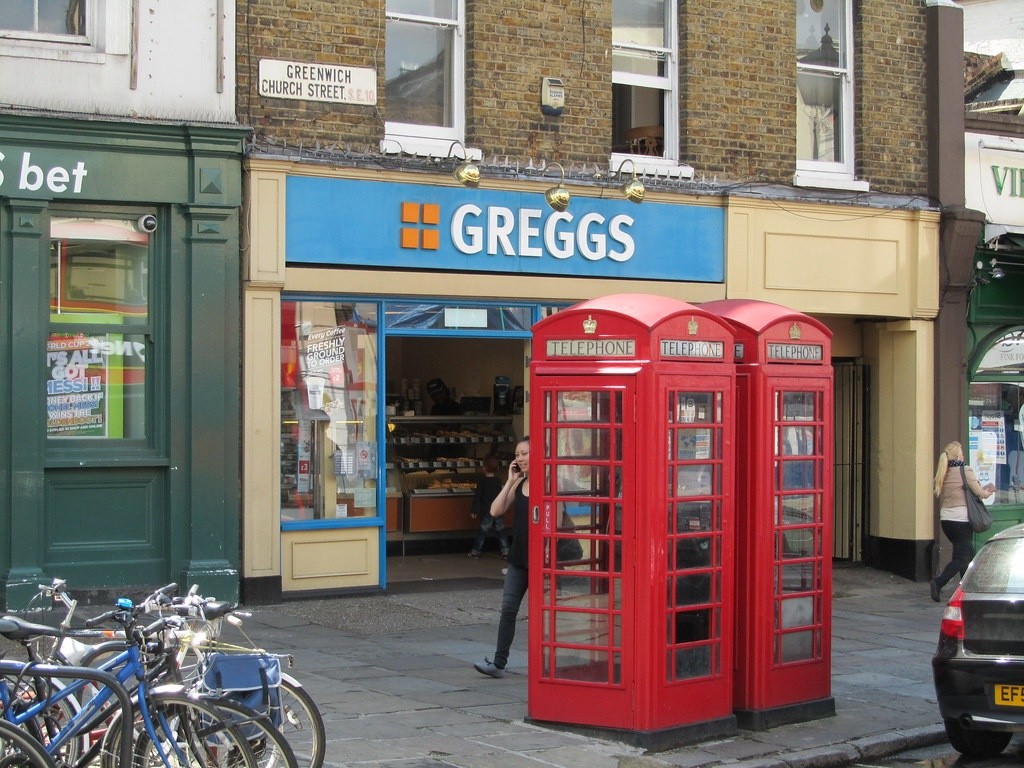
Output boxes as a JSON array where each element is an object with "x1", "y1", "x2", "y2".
[
  {"x1": 464, "y1": 457, "x2": 511, "y2": 560},
  {"x1": 473, "y1": 437, "x2": 567, "y2": 678},
  {"x1": 928, "y1": 441, "x2": 995, "y2": 603}
]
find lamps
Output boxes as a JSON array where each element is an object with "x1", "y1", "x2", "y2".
[
  {"x1": 617, "y1": 157, "x2": 645, "y2": 205},
  {"x1": 448, "y1": 138, "x2": 479, "y2": 188},
  {"x1": 990, "y1": 260, "x2": 1004, "y2": 280},
  {"x1": 524, "y1": 163, "x2": 571, "y2": 213}
]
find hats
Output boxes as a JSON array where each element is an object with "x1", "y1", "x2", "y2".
[{"x1": 426, "y1": 378, "x2": 444, "y2": 397}]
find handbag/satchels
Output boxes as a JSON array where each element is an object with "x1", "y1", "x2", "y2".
[
  {"x1": 960, "y1": 465, "x2": 993, "y2": 533},
  {"x1": 201, "y1": 654, "x2": 284, "y2": 747},
  {"x1": 556, "y1": 511, "x2": 583, "y2": 561}
]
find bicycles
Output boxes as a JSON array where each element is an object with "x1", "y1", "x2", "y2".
[{"x1": 0, "y1": 578, "x2": 326, "y2": 768}]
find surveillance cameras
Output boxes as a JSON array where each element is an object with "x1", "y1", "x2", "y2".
[{"x1": 138, "y1": 215, "x2": 158, "y2": 234}]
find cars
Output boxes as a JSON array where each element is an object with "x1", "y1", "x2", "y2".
[{"x1": 932, "y1": 523, "x2": 1024, "y2": 758}]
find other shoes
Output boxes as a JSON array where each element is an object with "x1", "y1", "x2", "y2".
[
  {"x1": 929, "y1": 578, "x2": 940, "y2": 602},
  {"x1": 474, "y1": 657, "x2": 505, "y2": 678},
  {"x1": 465, "y1": 552, "x2": 480, "y2": 560},
  {"x1": 502, "y1": 555, "x2": 509, "y2": 560}
]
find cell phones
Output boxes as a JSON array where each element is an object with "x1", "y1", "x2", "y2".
[{"x1": 512, "y1": 463, "x2": 521, "y2": 473}]
